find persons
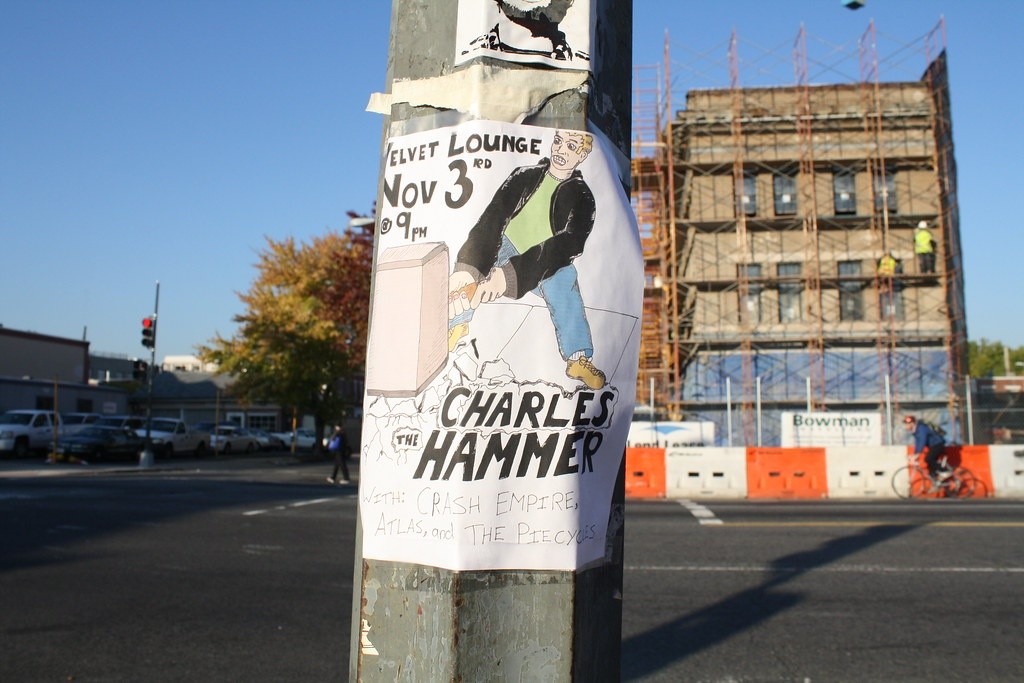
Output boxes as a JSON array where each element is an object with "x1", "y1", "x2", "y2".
[
  {"x1": 326, "y1": 423, "x2": 351, "y2": 485},
  {"x1": 877, "y1": 250, "x2": 901, "y2": 273},
  {"x1": 903, "y1": 416, "x2": 953, "y2": 493},
  {"x1": 912, "y1": 221, "x2": 936, "y2": 273}
]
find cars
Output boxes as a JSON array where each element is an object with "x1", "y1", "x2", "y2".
[
  {"x1": 48, "y1": 425, "x2": 141, "y2": 464},
  {"x1": 64, "y1": 412, "x2": 329, "y2": 460}
]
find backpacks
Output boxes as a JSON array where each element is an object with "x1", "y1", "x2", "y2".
[{"x1": 923, "y1": 422, "x2": 947, "y2": 436}]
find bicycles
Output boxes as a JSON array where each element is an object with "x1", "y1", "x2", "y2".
[{"x1": 891, "y1": 454, "x2": 976, "y2": 499}]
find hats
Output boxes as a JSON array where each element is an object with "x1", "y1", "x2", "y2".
[{"x1": 335, "y1": 421, "x2": 342, "y2": 426}]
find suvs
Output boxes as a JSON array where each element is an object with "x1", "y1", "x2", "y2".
[{"x1": 0, "y1": 410, "x2": 63, "y2": 459}]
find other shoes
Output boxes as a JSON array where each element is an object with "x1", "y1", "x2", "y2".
[
  {"x1": 938, "y1": 471, "x2": 953, "y2": 483},
  {"x1": 326, "y1": 477, "x2": 334, "y2": 484},
  {"x1": 924, "y1": 487, "x2": 939, "y2": 494},
  {"x1": 339, "y1": 480, "x2": 349, "y2": 484}
]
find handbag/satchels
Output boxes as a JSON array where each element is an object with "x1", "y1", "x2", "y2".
[{"x1": 329, "y1": 437, "x2": 340, "y2": 450}]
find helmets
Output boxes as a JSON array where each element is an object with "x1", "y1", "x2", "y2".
[
  {"x1": 903, "y1": 416, "x2": 915, "y2": 423},
  {"x1": 918, "y1": 222, "x2": 927, "y2": 228},
  {"x1": 892, "y1": 250, "x2": 899, "y2": 260}
]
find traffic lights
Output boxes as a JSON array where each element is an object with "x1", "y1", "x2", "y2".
[
  {"x1": 141, "y1": 316, "x2": 154, "y2": 348},
  {"x1": 133, "y1": 360, "x2": 147, "y2": 383}
]
